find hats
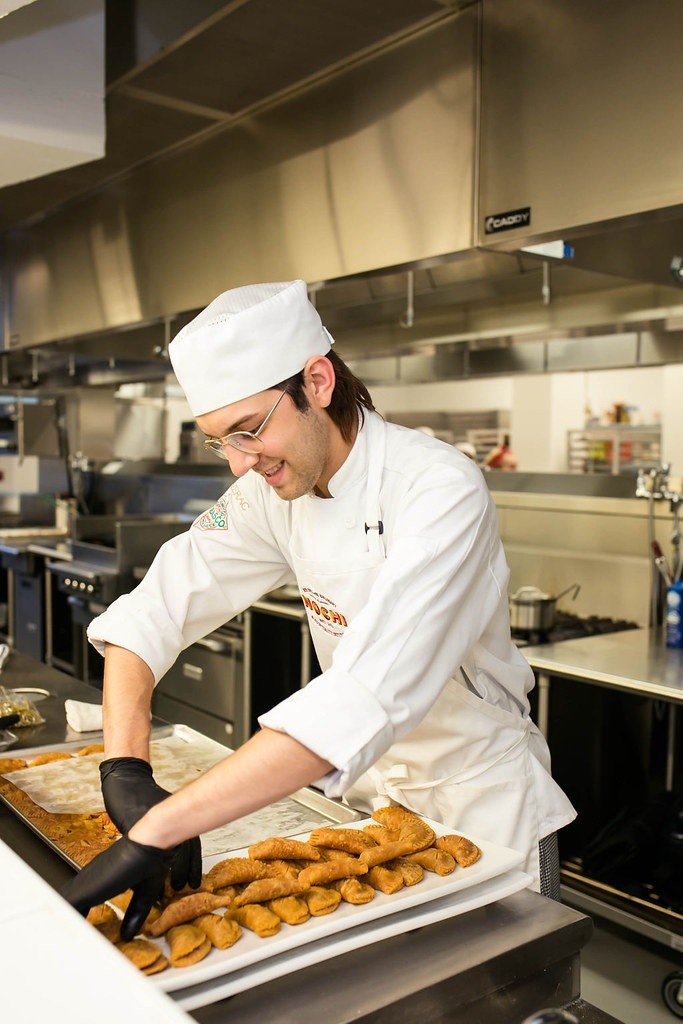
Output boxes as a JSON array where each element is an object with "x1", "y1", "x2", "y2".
[{"x1": 169, "y1": 280, "x2": 335, "y2": 417}]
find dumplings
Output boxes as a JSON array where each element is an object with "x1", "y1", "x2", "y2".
[
  {"x1": 82, "y1": 807, "x2": 481, "y2": 974},
  {"x1": 0, "y1": 742, "x2": 106, "y2": 776}
]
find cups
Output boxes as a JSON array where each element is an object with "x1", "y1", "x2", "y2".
[{"x1": 522, "y1": 1006, "x2": 578, "y2": 1024}]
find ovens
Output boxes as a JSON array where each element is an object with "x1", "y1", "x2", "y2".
[{"x1": 131, "y1": 564, "x2": 251, "y2": 751}]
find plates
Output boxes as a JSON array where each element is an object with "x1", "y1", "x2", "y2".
[{"x1": 92, "y1": 797, "x2": 536, "y2": 1013}]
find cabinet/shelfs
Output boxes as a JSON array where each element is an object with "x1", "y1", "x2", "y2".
[
  {"x1": 468, "y1": 428, "x2": 512, "y2": 473},
  {"x1": 568, "y1": 425, "x2": 663, "y2": 478}
]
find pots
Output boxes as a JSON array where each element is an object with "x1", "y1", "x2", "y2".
[{"x1": 509, "y1": 580, "x2": 581, "y2": 643}]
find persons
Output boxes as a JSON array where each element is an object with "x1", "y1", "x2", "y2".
[{"x1": 59, "y1": 280, "x2": 577, "y2": 939}]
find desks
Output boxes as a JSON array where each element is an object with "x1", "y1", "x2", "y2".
[{"x1": 521, "y1": 628, "x2": 683, "y2": 1021}]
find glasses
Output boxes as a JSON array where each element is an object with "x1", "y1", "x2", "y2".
[{"x1": 203, "y1": 382, "x2": 291, "y2": 460}]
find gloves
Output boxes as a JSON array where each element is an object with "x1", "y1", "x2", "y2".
[
  {"x1": 64, "y1": 828, "x2": 171, "y2": 943},
  {"x1": 99, "y1": 757, "x2": 203, "y2": 892}
]
